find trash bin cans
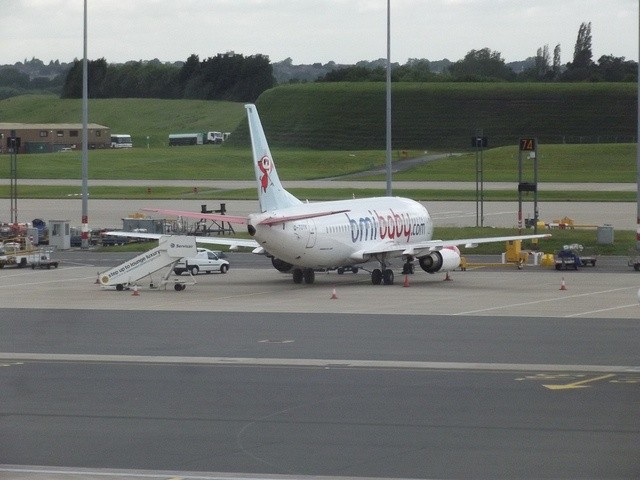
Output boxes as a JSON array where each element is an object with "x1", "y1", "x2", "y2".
[{"x1": 505, "y1": 239, "x2": 522, "y2": 263}]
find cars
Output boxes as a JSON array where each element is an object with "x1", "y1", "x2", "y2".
[{"x1": 176, "y1": 244, "x2": 231, "y2": 276}]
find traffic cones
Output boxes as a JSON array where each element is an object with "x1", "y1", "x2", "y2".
[
  {"x1": 128, "y1": 285, "x2": 141, "y2": 296},
  {"x1": 403, "y1": 274, "x2": 411, "y2": 287},
  {"x1": 559, "y1": 277, "x2": 568, "y2": 290},
  {"x1": 330, "y1": 286, "x2": 339, "y2": 300}
]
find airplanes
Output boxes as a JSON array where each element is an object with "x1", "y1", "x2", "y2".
[{"x1": 92, "y1": 94, "x2": 553, "y2": 289}]
